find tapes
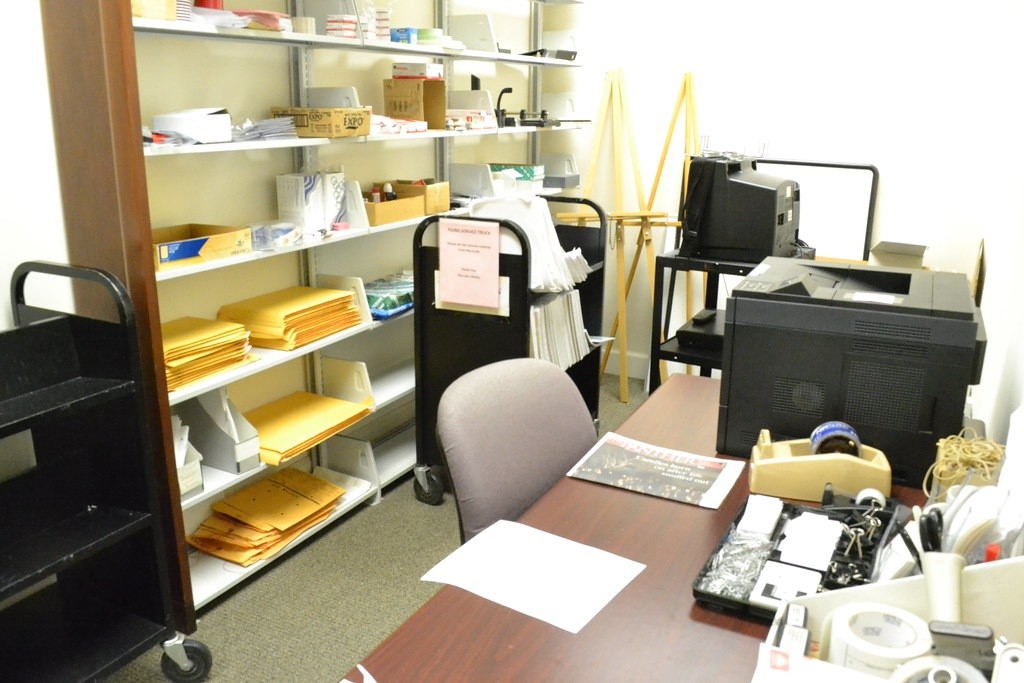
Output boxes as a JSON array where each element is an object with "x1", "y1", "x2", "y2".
[
  {"x1": 855, "y1": 489, "x2": 886, "y2": 508},
  {"x1": 829, "y1": 604, "x2": 990, "y2": 683}
]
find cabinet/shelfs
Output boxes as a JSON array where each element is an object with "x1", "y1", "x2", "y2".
[
  {"x1": 0, "y1": 260, "x2": 212, "y2": 682},
  {"x1": 648, "y1": 158, "x2": 879, "y2": 395},
  {"x1": 41, "y1": 1, "x2": 583, "y2": 611},
  {"x1": 412, "y1": 194, "x2": 607, "y2": 505}
]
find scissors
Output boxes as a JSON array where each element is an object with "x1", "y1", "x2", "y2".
[{"x1": 919, "y1": 508, "x2": 943, "y2": 552}]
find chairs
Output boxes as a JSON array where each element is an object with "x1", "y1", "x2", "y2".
[{"x1": 435, "y1": 359, "x2": 598, "y2": 546}]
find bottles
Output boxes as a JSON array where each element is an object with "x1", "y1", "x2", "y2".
[{"x1": 364, "y1": 183, "x2": 396, "y2": 203}]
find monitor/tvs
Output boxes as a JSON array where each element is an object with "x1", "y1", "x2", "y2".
[{"x1": 681, "y1": 153, "x2": 800, "y2": 265}]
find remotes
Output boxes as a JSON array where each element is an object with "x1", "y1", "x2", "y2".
[{"x1": 691, "y1": 310, "x2": 717, "y2": 324}]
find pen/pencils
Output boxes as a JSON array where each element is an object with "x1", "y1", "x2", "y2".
[{"x1": 896, "y1": 518, "x2": 923, "y2": 571}]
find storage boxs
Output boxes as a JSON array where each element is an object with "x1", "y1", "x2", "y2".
[
  {"x1": 152, "y1": 223, "x2": 252, "y2": 271},
  {"x1": 543, "y1": 175, "x2": 579, "y2": 188},
  {"x1": 362, "y1": 192, "x2": 424, "y2": 227},
  {"x1": 153, "y1": 107, "x2": 232, "y2": 143},
  {"x1": 391, "y1": 27, "x2": 418, "y2": 44},
  {"x1": 271, "y1": 106, "x2": 371, "y2": 138},
  {"x1": 373, "y1": 180, "x2": 450, "y2": 215},
  {"x1": 489, "y1": 163, "x2": 546, "y2": 180},
  {"x1": 178, "y1": 440, "x2": 204, "y2": 502},
  {"x1": 277, "y1": 169, "x2": 346, "y2": 233},
  {"x1": 383, "y1": 78, "x2": 447, "y2": 130},
  {"x1": 392, "y1": 63, "x2": 444, "y2": 79}
]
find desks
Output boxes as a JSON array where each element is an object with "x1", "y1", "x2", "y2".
[{"x1": 338, "y1": 373, "x2": 987, "y2": 683}]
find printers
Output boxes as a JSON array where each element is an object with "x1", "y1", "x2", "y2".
[{"x1": 715, "y1": 256, "x2": 987, "y2": 492}]
